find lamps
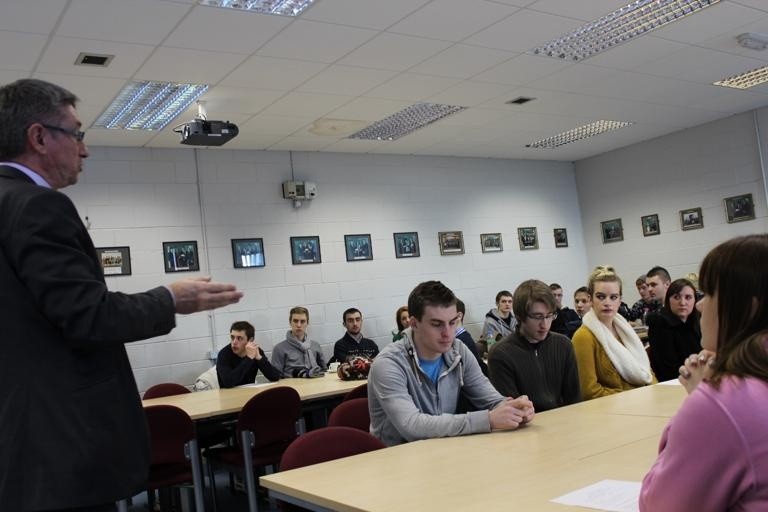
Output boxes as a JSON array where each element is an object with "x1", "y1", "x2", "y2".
[
  {"x1": 219, "y1": 386, "x2": 303, "y2": 512},
  {"x1": 279, "y1": 426, "x2": 387, "y2": 472},
  {"x1": 143, "y1": 383, "x2": 235, "y2": 489},
  {"x1": 142, "y1": 405, "x2": 218, "y2": 512},
  {"x1": 327, "y1": 383, "x2": 371, "y2": 433}
]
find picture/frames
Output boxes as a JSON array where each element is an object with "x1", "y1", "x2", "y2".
[
  {"x1": 140, "y1": 369, "x2": 368, "y2": 512},
  {"x1": 259, "y1": 377, "x2": 702, "y2": 512}
]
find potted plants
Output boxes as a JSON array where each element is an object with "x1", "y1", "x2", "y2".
[
  {"x1": 554, "y1": 228, "x2": 568, "y2": 248},
  {"x1": 600, "y1": 218, "x2": 624, "y2": 244},
  {"x1": 723, "y1": 193, "x2": 756, "y2": 224},
  {"x1": 480, "y1": 233, "x2": 503, "y2": 253},
  {"x1": 344, "y1": 234, "x2": 373, "y2": 262},
  {"x1": 231, "y1": 238, "x2": 265, "y2": 268},
  {"x1": 438, "y1": 231, "x2": 465, "y2": 256},
  {"x1": 290, "y1": 236, "x2": 321, "y2": 265},
  {"x1": 162, "y1": 240, "x2": 200, "y2": 273},
  {"x1": 679, "y1": 207, "x2": 704, "y2": 231},
  {"x1": 641, "y1": 213, "x2": 660, "y2": 237},
  {"x1": 393, "y1": 232, "x2": 420, "y2": 258},
  {"x1": 517, "y1": 227, "x2": 539, "y2": 250},
  {"x1": 94, "y1": 246, "x2": 131, "y2": 276}
]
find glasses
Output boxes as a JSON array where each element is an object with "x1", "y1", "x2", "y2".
[
  {"x1": 89, "y1": 80, "x2": 209, "y2": 132},
  {"x1": 196, "y1": 0, "x2": 317, "y2": 17},
  {"x1": 525, "y1": 119, "x2": 633, "y2": 149},
  {"x1": 712, "y1": 64, "x2": 768, "y2": 90},
  {"x1": 524, "y1": 0, "x2": 721, "y2": 63},
  {"x1": 348, "y1": 103, "x2": 464, "y2": 141}
]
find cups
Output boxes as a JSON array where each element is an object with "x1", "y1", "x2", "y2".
[
  {"x1": 40, "y1": 123, "x2": 86, "y2": 144},
  {"x1": 525, "y1": 311, "x2": 559, "y2": 323}
]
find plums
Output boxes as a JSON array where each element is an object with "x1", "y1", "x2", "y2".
[{"x1": 173, "y1": 118, "x2": 238, "y2": 146}]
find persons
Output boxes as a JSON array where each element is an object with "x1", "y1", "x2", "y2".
[
  {"x1": 240, "y1": 248, "x2": 264, "y2": 266},
  {"x1": 645, "y1": 279, "x2": 702, "y2": 382},
  {"x1": 333, "y1": 309, "x2": 380, "y2": 362},
  {"x1": 104, "y1": 255, "x2": 122, "y2": 267},
  {"x1": 626, "y1": 276, "x2": 653, "y2": 328},
  {"x1": 457, "y1": 298, "x2": 486, "y2": 371},
  {"x1": 645, "y1": 266, "x2": 671, "y2": 307},
  {"x1": 272, "y1": 306, "x2": 326, "y2": 382},
  {"x1": 367, "y1": 282, "x2": 535, "y2": 444},
  {"x1": 391, "y1": 307, "x2": 412, "y2": 340},
  {"x1": 571, "y1": 265, "x2": 658, "y2": 403},
  {"x1": 217, "y1": 322, "x2": 280, "y2": 388},
  {"x1": 550, "y1": 283, "x2": 580, "y2": 340},
  {"x1": 488, "y1": 279, "x2": 580, "y2": 415},
  {"x1": 557, "y1": 286, "x2": 593, "y2": 338},
  {"x1": 637, "y1": 235, "x2": 768, "y2": 511},
  {"x1": 166, "y1": 247, "x2": 196, "y2": 267},
  {"x1": 686, "y1": 215, "x2": 699, "y2": 224},
  {"x1": 0, "y1": 77, "x2": 247, "y2": 510},
  {"x1": 482, "y1": 290, "x2": 518, "y2": 347}
]
